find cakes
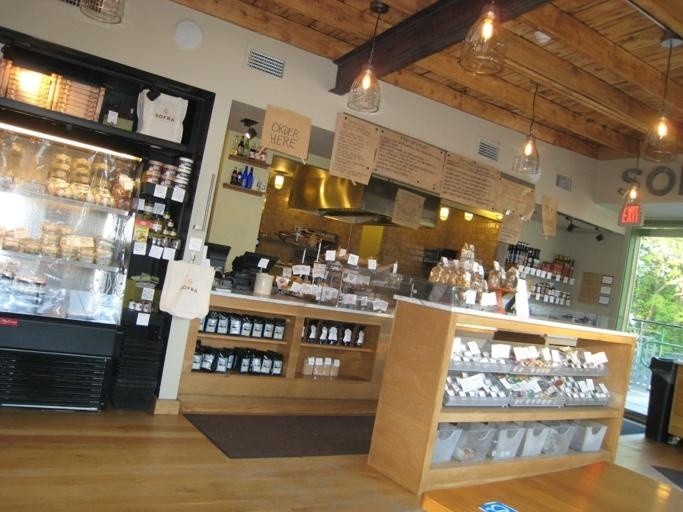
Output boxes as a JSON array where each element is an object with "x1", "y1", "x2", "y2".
[{"x1": 0, "y1": 255, "x2": 47, "y2": 292}]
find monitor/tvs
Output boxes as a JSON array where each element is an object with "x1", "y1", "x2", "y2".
[
  {"x1": 204, "y1": 241, "x2": 231, "y2": 273},
  {"x1": 232, "y1": 252, "x2": 278, "y2": 277}
]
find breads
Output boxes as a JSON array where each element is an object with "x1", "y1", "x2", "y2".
[{"x1": 0, "y1": 126, "x2": 134, "y2": 264}]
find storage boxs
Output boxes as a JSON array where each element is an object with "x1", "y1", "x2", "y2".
[{"x1": 433, "y1": 421, "x2": 607, "y2": 464}]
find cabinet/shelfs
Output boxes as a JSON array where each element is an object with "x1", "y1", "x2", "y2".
[
  {"x1": 223, "y1": 154, "x2": 269, "y2": 197},
  {"x1": 0, "y1": 25, "x2": 216, "y2": 337},
  {"x1": 506, "y1": 263, "x2": 570, "y2": 303},
  {"x1": 367, "y1": 294, "x2": 640, "y2": 494},
  {"x1": 292, "y1": 307, "x2": 393, "y2": 398},
  {"x1": 180, "y1": 295, "x2": 299, "y2": 400}
]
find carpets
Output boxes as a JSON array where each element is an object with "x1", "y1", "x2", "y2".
[{"x1": 184, "y1": 414, "x2": 375, "y2": 459}]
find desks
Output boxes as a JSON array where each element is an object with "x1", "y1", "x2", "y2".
[{"x1": 421, "y1": 461, "x2": 683, "y2": 512}]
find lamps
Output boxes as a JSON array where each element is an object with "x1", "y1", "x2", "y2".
[
  {"x1": 596, "y1": 228, "x2": 604, "y2": 242},
  {"x1": 513, "y1": 80, "x2": 539, "y2": 174},
  {"x1": 80, "y1": 0, "x2": 126, "y2": 23},
  {"x1": 642, "y1": 33, "x2": 683, "y2": 162},
  {"x1": 274, "y1": 169, "x2": 288, "y2": 190},
  {"x1": 621, "y1": 155, "x2": 643, "y2": 206},
  {"x1": 458, "y1": 0, "x2": 506, "y2": 76},
  {"x1": 347, "y1": 1, "x2": 389, "y2": 114},
  {"x1": 566, "y1": 216, "x2": 576, "y2": 231}
]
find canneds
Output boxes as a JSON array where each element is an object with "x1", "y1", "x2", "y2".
[{"x1": 530, "y1": 281, "x2": 570, "y2": 300}]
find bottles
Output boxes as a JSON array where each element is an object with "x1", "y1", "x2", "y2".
[
  {"x1": 230, "y1": 135, "x2": 267, "y2": 163},
  {"x1": 230, "y1": 165, "x2": 254, "y2": 189},
  {"x1": 505, "y1": 240, "x2": 541, "y2": 269},
  {"x1": 551, "y1": 254, "x2": 575, "y2": 278}
]
